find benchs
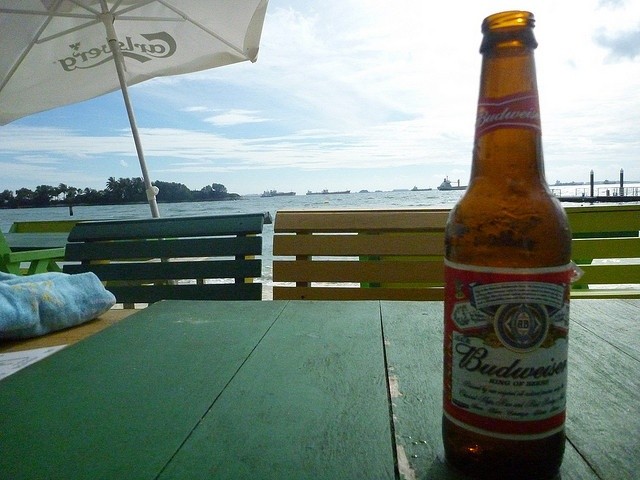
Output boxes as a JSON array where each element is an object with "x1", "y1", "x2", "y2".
[
  {"x1": 272, "y1": 209, "x2": 451, "y2": 302},
  {"x1": 62, "y1": 212, "x2": 273, "y2": 301},
  {"x1": 564, "y1": 204, "x2": 640, "y2": 299}
]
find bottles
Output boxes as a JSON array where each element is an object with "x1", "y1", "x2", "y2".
[{"x1": 442, "y1": 10, "x2": 573, "y2": 479}]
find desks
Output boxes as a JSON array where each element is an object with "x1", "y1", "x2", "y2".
[
  {"x1": 0, "y1": 300, "x2": 639, "y2": 478},
  {"x1": 0, "y1": 310, "x2": 143, "y2": 381}
]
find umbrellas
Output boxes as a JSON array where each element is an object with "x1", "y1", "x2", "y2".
[{"x1": 0, "y1": 0, "x2": 268, "y2": 286}]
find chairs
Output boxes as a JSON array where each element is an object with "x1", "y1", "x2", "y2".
[{"x1": 0, "y1": 231, "x2": 67, "y2": 276}]
